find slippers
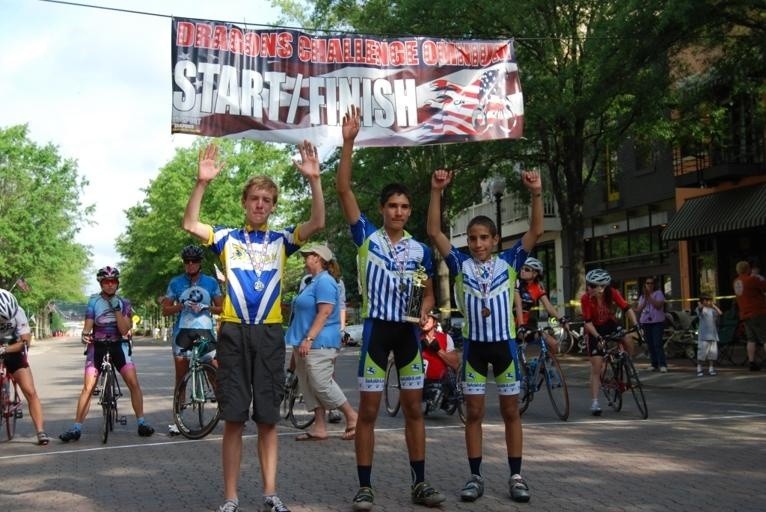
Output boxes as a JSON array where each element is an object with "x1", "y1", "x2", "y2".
[
  {"x1": 340, "y1": 425, "x2": 356, "y2": 441},
  {"x1": 295, "y1": 432, "x2": 330, "y2": 441}
]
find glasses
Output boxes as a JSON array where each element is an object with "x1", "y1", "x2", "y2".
[
  {"x1": 646, "y1": 281, "x2": 655, "y2": 285},
  {"x1": 100, "y1": 277, "x2": 119, "y2": 285},
  {"x1": 183, "y1": 258, "x2": 203, "y2": 264},
  {"x1": 521, "y1": 266, "x2": 531, "y2": 272},
  {"x1": 586, "y1": 282, "x2": 597, "y2": 289}
]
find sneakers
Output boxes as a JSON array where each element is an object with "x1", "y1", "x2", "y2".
[
  {"x1": 351, "y1": 486, "x2": 375, "y2": 512},
  {"x1": 659, "y1": 366, "x2": 668, "y2": 373},
  {"x1": 138, "y1": 422, "x2": 155, "y2": 438},
  {"x1": 696, "y1": 367, "x2": 704, "y2": 377},
  {"x1": 647, "y1": 366, "x2": 658, "y2": 371},
  {"x1": 590, "y1": 400, "x2": 603, "y2": 416},
  {"x1": 168, "y1": 423, "x2": 182, "y2": 436},
  {"x1": 37, "y1": 431, "x2": 50, "y2": 446},
  {"x1": 328, "y1": 409, "x2": 344, "y2": 424},
  {"x1": 58, "y1": 427, "x2": 82, "y2": 443},
  {"x1": 460, "y1": 473, "x2": 485, "y2": 502},
  {"x1": 217, "y1": 498, "x2": 241, "y2": 512},
  {"x1": 262, "y1": 493, "x2": 291, "y2": 512},
  {"x1": 707, "y1": 368, "x2": 717, "y2": 376},
  {"x1": 508, "y1": 473, "x2": 531, "y2": 503},
  {"x1": 749, "y1": 362, "x2": 760, "y2": 372},
  {"x1": 411, "y1": 479, "x2": 447, "y2": 506}
]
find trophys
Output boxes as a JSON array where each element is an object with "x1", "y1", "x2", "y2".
[{"x1": 402, "y1": 265, "x2": 429, "y2": 323}]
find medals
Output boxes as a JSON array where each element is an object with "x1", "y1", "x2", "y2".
[
  {"x1": 398, "y1": 282, "x2": 408, "y2": 292},
  {"x1": 254, "y1": 279, "x2": 264, "y2": 291}
]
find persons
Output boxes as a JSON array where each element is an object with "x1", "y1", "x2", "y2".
[
  {"x1": 420, "y1": 255, "x2": 766, "y2": 417},
  {"x1": 163, "y1": 244, "x2": 223, "y2": 435},
  {"x1": 285, "y1": 253, "x2": 346, "y2": 424},
  {"x1": 337, "y1": 105, "x2": 445, "y2": 512},
  {"x1": 59, "y1": 266, "x2": 154, "y2": 442},
  {"x1": 428, "y1": 170, "x2": 544, "y2": 502},
  {"x1": 284, "y1": 244, "x2": 359, "y2": 441},
  {"x1": 0, "y1": 288, "x2": 49, "y2": 444},
  {"x1": 181, "y1": 139, "x2": 326, "y2": 512}
]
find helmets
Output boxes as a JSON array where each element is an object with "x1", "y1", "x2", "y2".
[
  {"x1": 585, "y1": 268, "x2": 612, "y2": 287},
  {"x1": 181, "y1": 244, "x2": 205, "y2": 259},
  {"x1": 427, "y1": 307, "x2": 442, "y2": 321},
  {"x1": 524, "y1": 256, "x2": 544, "y2": 276},
  {"x1": 96, "y1": 266, "x2": 120, "y2": 282},
  {"x1": 0, "y1": 287, "x2": 20, "y2": 322}
]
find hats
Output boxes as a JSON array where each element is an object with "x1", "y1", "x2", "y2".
[
  {"x1": 698, "y1": 291, "x2": 715, "y2": 299},
  {"x1": 298, "y1": 245, "x2": 334, "y2": 264}
]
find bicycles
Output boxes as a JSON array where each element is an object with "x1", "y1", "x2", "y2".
[{"x1": 567, "y1": 311, "x2": 751, "y2": 366}]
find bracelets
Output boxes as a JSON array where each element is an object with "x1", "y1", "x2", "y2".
[
  {"x1": 305, "y1": 336, "x2": 315, "y2": 341},
  {"x1": 532, "y1": 193, "x2": 542, "y2": 197}
]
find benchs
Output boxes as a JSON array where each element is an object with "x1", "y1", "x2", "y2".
[{"x1": 688, "y1": 311, "x2": 749, "y2": 366}]
